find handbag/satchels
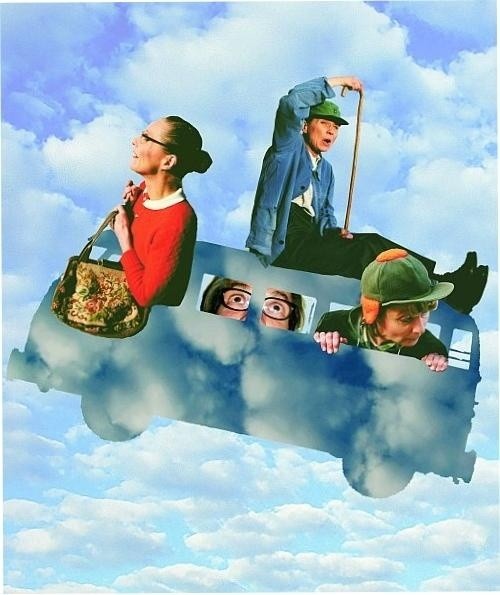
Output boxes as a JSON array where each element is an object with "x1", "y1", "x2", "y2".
[{"x1": 52, "y1": 205, "x2": 156, "y2": 339}]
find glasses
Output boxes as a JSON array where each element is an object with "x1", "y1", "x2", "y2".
[
  {"x1": 140, "y1": 130, "x2": 172, "y2": 151},
  {"x1": 217, "y1": 286, "x2": 299, "y2": 322}
]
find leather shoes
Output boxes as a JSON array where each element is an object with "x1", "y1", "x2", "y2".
[
  {"x1": 443, "y1": 251, "x2": 477, "y2": 283},
  {"x1": 446, "y1": 263, "x2": 488, "y2": 316}
]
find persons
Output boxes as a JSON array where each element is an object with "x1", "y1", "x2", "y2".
[
  {"x1": 200, "y1": 275, "x2": 307, "y2": 333},
  {"x1": 242, "y1": 75, "x2": 489, "y2": 318},
  {"x1": 108, "y1": 115, "x2": 213, "y2": 307},
  {"x1": 311, "y1": 247, "x2": 449, "y2": 372}
]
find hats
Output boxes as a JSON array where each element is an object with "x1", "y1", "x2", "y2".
[
  {"x1": 360, "y1": 247, "x2": 456, "y2": 325},
  {"x1": 308, "y1": 99, "x2": 350, "y2": 128}
]
